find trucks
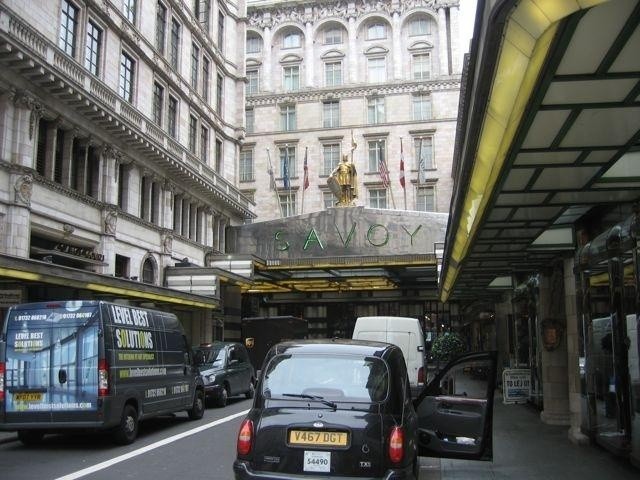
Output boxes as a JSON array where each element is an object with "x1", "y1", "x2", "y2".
[
  {"x1": 0, "y1": 300, "x2": 205, "y2": 445},
  {"x1": 586, "y1": 313, "x2": 639, "y2": 417},
  {"x1": 351, "y1": 316, "x2": 428, "y2": 400}
]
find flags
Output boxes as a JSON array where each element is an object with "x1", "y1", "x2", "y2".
[
  {"x1": 379, "y1": 145, "x2": 389, "y2": 189},
  {"x1": 266, "y1": 151, "x2": 277, "y2": 192},
  {"x1": 419, "y1": 140, "x2": 425, "y2": 185},
  {"x1": 283, "y1": 148, "x2": 289, "y2": 189},
  {"x1": 303, "y1": 150, "x2": 309, "y2": 189},
  {"x1": 399, "y1": 140, "x2": 405, "y2": 189}
]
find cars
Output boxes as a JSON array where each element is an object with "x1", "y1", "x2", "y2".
[
  {"x1": 233, "y1": 339, "x2": 497, "y2": 479},
  {"x1": 193, "y1": 342, "x2": 255, "y2": 406}
]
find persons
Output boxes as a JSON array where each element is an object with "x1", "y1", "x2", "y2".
[{"x1": 329, "y1": 153, "x2": 359, "y2": 207}]
